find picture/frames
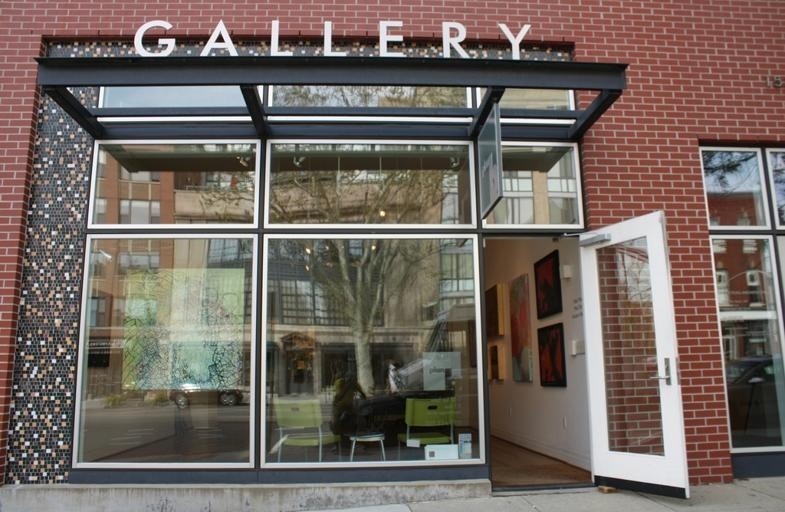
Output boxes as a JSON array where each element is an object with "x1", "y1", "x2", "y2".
[{"x1": 484, "y1": 248, "x2": 569, "y2": 390}]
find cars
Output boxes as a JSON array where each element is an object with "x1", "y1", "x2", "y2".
[{"x1": 168, "y1": 380, "x2": 244, "y2": 409}]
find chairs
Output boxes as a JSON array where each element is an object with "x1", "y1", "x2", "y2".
[
  {"x1": 269, "y1": 389, "x2": 342, "y2": 462},
  {"x1": 395, "y1": 397, "x2": 457, "y2": 461}
]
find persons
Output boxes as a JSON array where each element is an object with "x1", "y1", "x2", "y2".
[{"x1": 329, "y1": 367, "x2": 367, "y2": 462}]
